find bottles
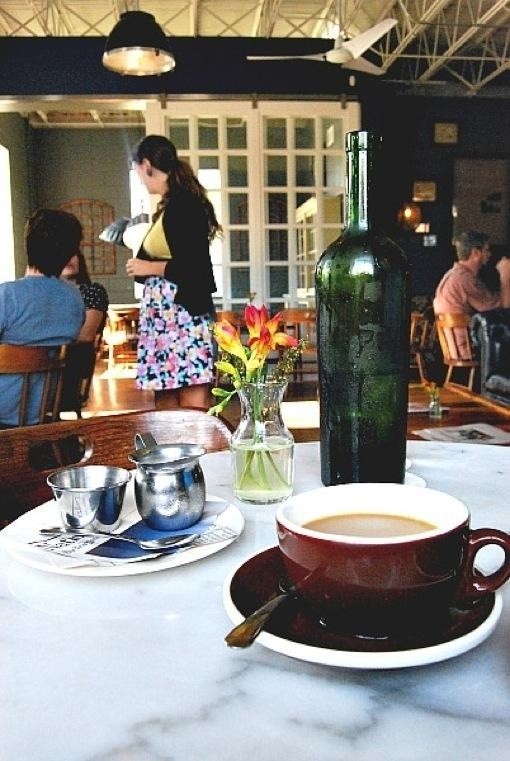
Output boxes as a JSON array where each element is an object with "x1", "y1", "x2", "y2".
[{"x1": 315, "y1": 129, "x2": 414, "y2": 487}]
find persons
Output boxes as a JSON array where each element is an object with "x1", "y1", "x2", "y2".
[
  {"x1": 124, "y1": 134, "x2": 226, "y2": 410},
  {"x1": 61, "y1": 250, "x2": 110, "y2": 420},
  {"x1": 432, "y1": 229, "x2": 510, "y2": 397},
  {"x1": 0, "y1": 210, "x2": 86, "y2": 431}
]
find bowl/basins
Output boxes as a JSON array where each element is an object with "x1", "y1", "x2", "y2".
[{"x1": 47, "y1": 465, "x2": 133, "y2": 534}]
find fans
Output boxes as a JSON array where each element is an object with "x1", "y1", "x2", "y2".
[{"x1": 246, "y1": 0, "x2": 399, "y2": 76}]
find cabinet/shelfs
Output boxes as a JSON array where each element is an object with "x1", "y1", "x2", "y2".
[{"x1": 296, "y1": 195, "x2": 342, "y2": 298}]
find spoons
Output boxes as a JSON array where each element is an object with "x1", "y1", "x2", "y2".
[{"x1": 40, "y1": 527, "x2": 200, "y2": 553}]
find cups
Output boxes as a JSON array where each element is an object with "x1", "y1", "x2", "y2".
[
  {"x1": 128, "y1": 432, "x2": 209, "y2": 532},
  {"x1": 277, "y1": 482, "x2": 510, "y2": 639}
]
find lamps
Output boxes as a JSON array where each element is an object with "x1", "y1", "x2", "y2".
[{"x1": 101, "y1": 10, "x2": 176, "y2": 76}]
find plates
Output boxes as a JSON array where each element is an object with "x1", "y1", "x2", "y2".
[
  {"x1": 7, "y1": 493, "x2": 248, "y2": 578},
  {"x1": 222, "y1": 544, "x2": 503, "y2": 669}
]
